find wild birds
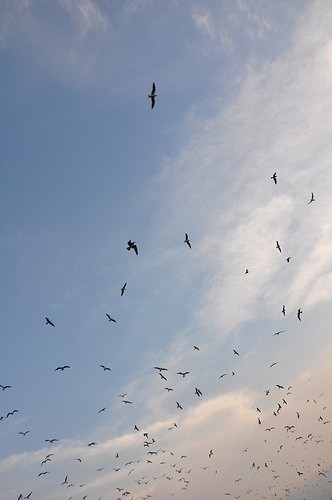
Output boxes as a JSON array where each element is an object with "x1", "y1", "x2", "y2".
[
  {"x1": 1, "y1": 368, "x2": 332, "y2": 500},
  {"x1": 297, "y1": 308, "x2": 302, "y2": 321},
  {"x1": 147, "y1": 83, "x2": 158, "y2": 111},
  {"x1": 275, "y1": 240, "x2": 282, "y2": 252},
  {"x1": 99, "y1": 365, "x2": 112, "y2": 372},
  {"x1": 54, "y1": 366, "x2": 71, "y2": 372},
  {"x1": 286, "y1": 257, "x2": 291, "y2": 262},
  {"x1": 270, "y1": 361, "x2": 280, "y2": 368},
  {"x1": 6, "y1": 409, "x2": 19, "y2": 417},
  {"x1": 232, "y1": 350, "x2": 244, "y2": 356},
  {"x1": 282, "y1": 305, "x2": 286, "y2": 316},
  {"x1": 193, "y1": 345, "x2": 200, "y2": 350},
  {"x1": 245, "y1": 269, "x2": 250, "y2": 275},
  {"x1": 271, "y1": 330, "x2": 286, "y2": 336},
  {"x1": 184, "y1": 233, "x2": 193, "y2": 249},
  {"x1": 106, "y1": 314, "x2": 117, "y2": 323},
  {"x1": 45, "y1": 317, "x2": 55, "y2": 327},
  {"x1": 126, "y1": 240, "x2": 139, "y2": 256},
  {"x1": 0, "y1": 385, "x2": 12, "y2": 391},
  {"x1": 270, "y1": 172, "x2": 278, "y2": 184},
  {"x1": 120, "y1": 282, "x2": 128, "y2": 296},
  {"x1": 308, "y1": 192, "x2": 315, "y2": 205}
]
